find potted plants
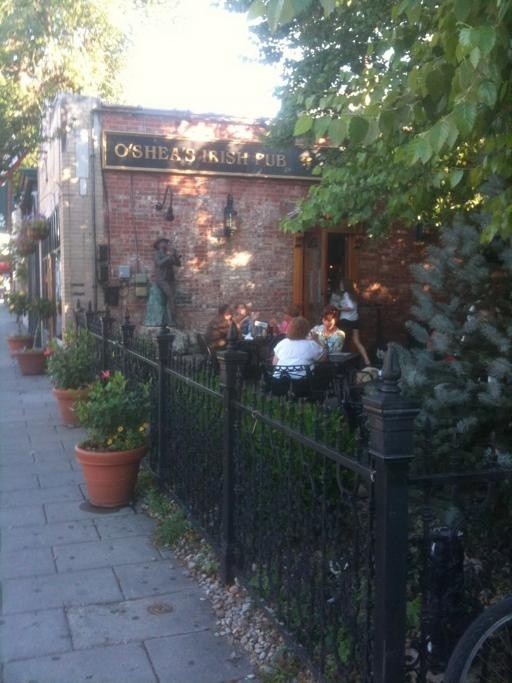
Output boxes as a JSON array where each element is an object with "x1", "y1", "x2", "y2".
[
  {"x1": 10, "y1": 296, "x2": 58, "y2": 374},
  {"x1": 3, "y1": 289, "x2": 32, "y2": 357}
]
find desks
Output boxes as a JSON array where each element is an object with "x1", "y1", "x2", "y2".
[
  {"x1": 227, "y1": 336, "x2": 278, "y2": 381},
  {"x1": 322, "y1": 347, "x2": 361, "y2": 395}
]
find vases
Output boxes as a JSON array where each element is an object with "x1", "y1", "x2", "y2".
[
  {"x1": 18, "y1": 242, "x2": 33, "y2": 251},
  {"x1": 47, "y1": 381, "x2": 99, "y2": 428},
  {"x1": 74, "y1": 435, "x2": 148, "y2": 509},
  {"x1": 25, "y1": 226, "x2": 38, "y2": 239}
]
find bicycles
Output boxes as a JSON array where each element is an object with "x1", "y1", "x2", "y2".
[{"x1": 442, "y1": 598, "x2": 512, "y2": 682}]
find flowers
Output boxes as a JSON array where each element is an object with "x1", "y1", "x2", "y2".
[
  {"x1": 41, "y1": 322, "x2": 104, "y2": 387},
  {"x1": 12, "y1": 235, "x2": 34, "y2": 244},
  {"x1": 70, "y1": 367, "x2": 166, "y2": 449},
  {"x1": 20, "y1": 210, "x2": 50, "y2": 228}
]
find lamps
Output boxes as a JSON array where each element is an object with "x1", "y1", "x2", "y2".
[
  {"x1": 223, "y1": 193, "x2": 240, "y2": 236},
  {"x1": 155, "y1": 183, "x2": 175, "y2": 221}
]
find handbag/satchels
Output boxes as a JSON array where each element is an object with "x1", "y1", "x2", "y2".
[
  {"x1": 262, "y1": 375, "x2": 290, "y2": 397},
  {"x1": 357, "y1": 367, "x2": 379, "y2": 382}
]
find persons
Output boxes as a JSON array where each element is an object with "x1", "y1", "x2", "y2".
[
  {"x1": 272, "y1": 314, "x2": 329, "y2": 401},
  {"x1": 202, "y1": 302, "x2": 245, "y2": 348},
  {"x1": 231, "y1": 299, "x2": 262, "y2": 334},
  {"x1": 307, "y1": 303, "x2": 346, "y2": 353},
  {"x1": 334, "y1": 278, "x2": 371, "y2": 365},
  {"x1": 267, "y1": 304, "x2": 303, "y2": 336},
  {"x1": 148, "y1": 232, "x2": 181, "y2": 326}
]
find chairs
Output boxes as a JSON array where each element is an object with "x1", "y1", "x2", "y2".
[
  {"x1": 264, "y1": 363, "x2": 317, "y2": 402},
  {"x1": 194, "y1": 328, "x2": 218, "y2": 370},
  {"x1": 258, "y1": 332, "x2": 284, "y2": 370}
]
